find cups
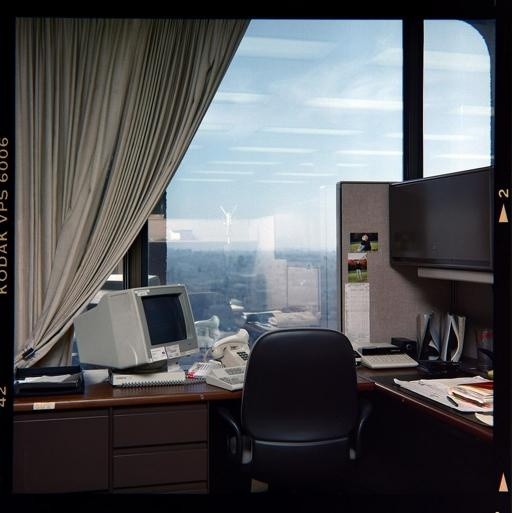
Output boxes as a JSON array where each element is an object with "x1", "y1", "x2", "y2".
[{"x1": 473, "y1": 327, "x2": 493, "y2": 370}]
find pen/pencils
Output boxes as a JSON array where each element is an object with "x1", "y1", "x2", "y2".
[{"x1": 447, "y1": 396, "x2": 458, "y2": 407}]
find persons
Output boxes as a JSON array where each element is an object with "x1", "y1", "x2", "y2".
[
  {"x1": 355, "y1": 261, "x2": 363, "y2": 282},
  {"x1": 357, "y1": 234, "x2": 372, "y2": 252}
]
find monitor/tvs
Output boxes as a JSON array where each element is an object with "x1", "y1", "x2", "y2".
[{"x1": 73, "y1": 283, "x2": 200, "y2": 371}]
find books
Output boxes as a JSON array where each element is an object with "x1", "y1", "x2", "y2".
[
  {"x1": 449, "y1": 382, "x2": 493, "y2": 409},
  {"x1": 416, "y1": 312, "x2": 480, "y2": 362},
  {"x1": 186, "y1": 362, "x2": 224, "y2": 380},
  {"x1": 267, "y1": 311, "x2": 318, "y2": 328}
]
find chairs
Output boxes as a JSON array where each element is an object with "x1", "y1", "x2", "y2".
[{"x1": 219, "y1": 329, "x2": 373, "y2": 494}]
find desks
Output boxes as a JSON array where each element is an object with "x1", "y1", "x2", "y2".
[
  {"x1": 14, "y1": 376, "x2": 375, "y2": 492},
  {"x1": 356, "y1": 362, "x2": 494, "y2": 493}
]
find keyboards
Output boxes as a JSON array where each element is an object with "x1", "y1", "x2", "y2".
[{"x1": 206, "y1": 366, "x2": 245, "y2": 391}]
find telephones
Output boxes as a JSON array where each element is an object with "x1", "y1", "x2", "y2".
[{"x1": 210, "y1": 328, "x2": 251, "y2": 367}]
